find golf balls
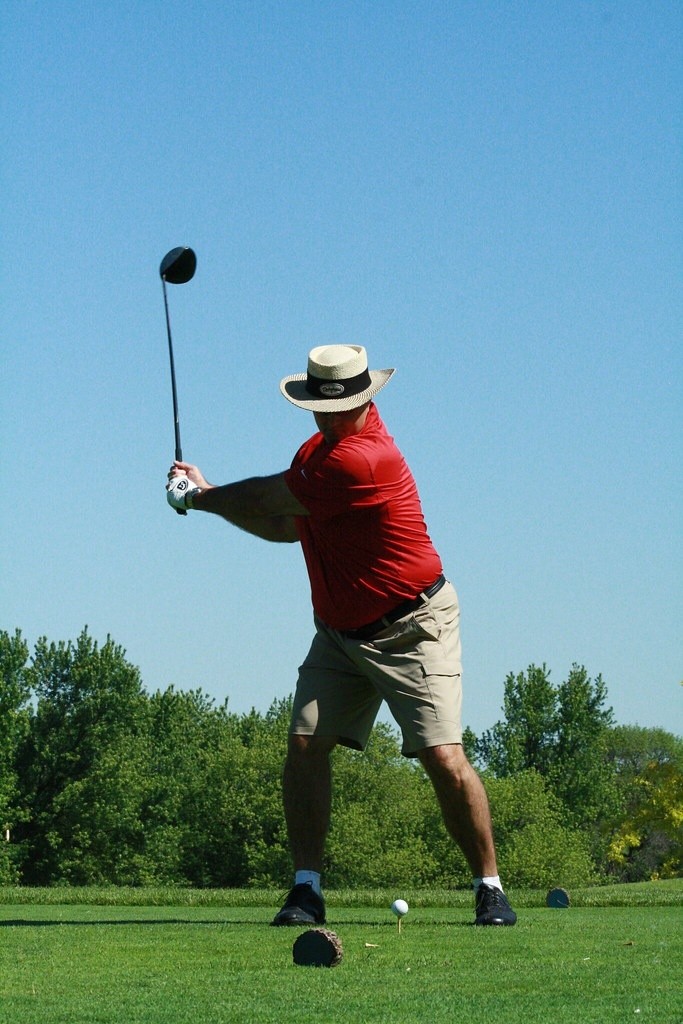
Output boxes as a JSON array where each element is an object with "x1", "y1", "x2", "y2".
[{"x1": 391, "y1": 899, "x2": 408, "y2": 916}]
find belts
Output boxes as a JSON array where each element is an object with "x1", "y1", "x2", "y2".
[{"x1": 343, "y1": 574, "x2": 446, "y2": 641}]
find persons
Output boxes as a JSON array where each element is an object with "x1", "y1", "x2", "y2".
[{"x1": 164, "y1": 342, "x2": 522, "y2": 931}]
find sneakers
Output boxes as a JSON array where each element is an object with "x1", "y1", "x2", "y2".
[
  {"x1": 473, "y1": 882, "x2": 517, "y2": 926},
  {"x1": 273, "y1": 880, "x2": 327, "y2": 926}
]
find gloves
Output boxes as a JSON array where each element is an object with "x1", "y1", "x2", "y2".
[{"x1": 166, "y1": 476, "x2": 201, "y2": 516}]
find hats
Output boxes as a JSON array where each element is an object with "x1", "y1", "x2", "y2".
[{"x1": 280, "y1": 344, "x2": 396, "y2": 413}]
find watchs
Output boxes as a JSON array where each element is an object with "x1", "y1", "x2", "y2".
[{"x1": 184, "y1": 485, "x2": 204, "y2": 509}]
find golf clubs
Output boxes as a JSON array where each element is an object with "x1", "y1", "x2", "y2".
[{"x1": 158, "y1": 246, "x2": 193, "y2": 516}]
